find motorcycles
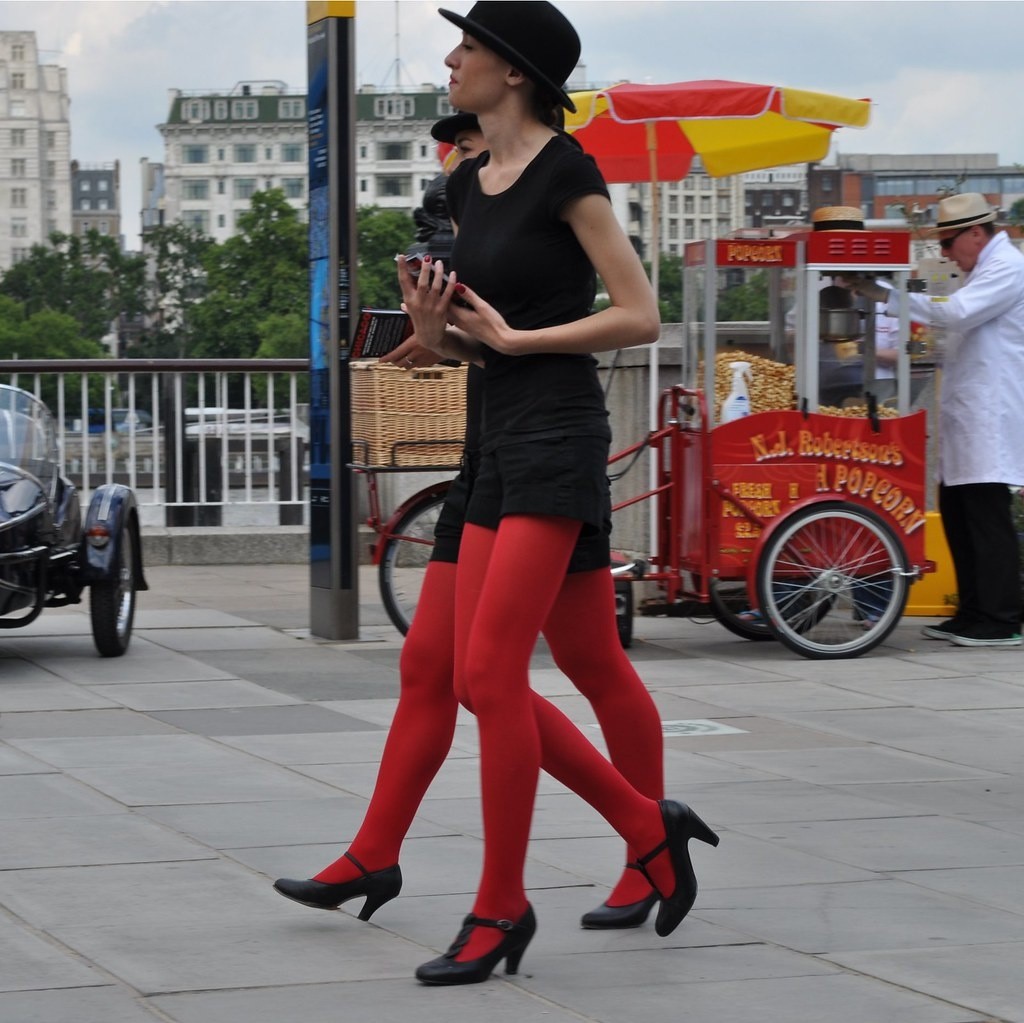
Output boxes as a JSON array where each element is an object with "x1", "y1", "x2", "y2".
[{"x1": 2, "y1": 386, "x2": 149, "y2": 658}]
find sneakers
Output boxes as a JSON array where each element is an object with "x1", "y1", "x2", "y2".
[{"x1": 922, "y1": 618, "x2": 1023, "y2": 647}]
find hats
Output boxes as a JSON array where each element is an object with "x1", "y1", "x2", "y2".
[
  {"x1": 431, "y1": 106, "x2": 581, "y2": 150},
  {"x1": 927, "y1": 191, "x2": 998, "y2": 231},
  {"x1": 806, "y1": 207, "x2": 871, "y2": 233},
  {"x1": 432, "y1": 2, "x2": 584, "y2": 116}
]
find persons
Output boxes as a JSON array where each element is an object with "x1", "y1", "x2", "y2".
[
  {"x1": 272, "y1": 112, "x2": 685, "y2": 932},
  {"x1": 786, "y1": 207, "x2": 912, "y2": 382},
  {"x1": 842, "y1": 274, "x2": 900, "y2": 379},
  {"x1": 402, "y1": 0, "x2": 723, "y2": 985},
  {"x1": 852, "y1": 191, "x2": 1022, "y2": 650}
]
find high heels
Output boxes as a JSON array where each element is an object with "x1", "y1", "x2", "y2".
[
  {"x1": 270, "y1": 851, "x2": 403, "y2": 921},
  {"x1": 579, "y1": 864, "x2": 657, "y2": 928},
  {"x1": 640, "y1": 797, "x2": 720, "y2": 936},
  {"x1": 415, "y1": 901, "x2": 536, "y2": 985}
]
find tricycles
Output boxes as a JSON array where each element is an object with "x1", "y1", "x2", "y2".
[{"x1": 364, "y1": 227, "x2": 926, "y2": 658}]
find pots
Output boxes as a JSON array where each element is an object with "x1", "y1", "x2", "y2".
[{"x1": 819, "y1": 306, "x2": 899, "y2": 342}]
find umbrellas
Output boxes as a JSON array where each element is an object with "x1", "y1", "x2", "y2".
[{"x1": 413, "y1": 76, "x2": 876, "y2": 426}]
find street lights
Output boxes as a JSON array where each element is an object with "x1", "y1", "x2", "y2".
[{"x1": 140, "y1": 206, "x2": 167, "y2": 318}]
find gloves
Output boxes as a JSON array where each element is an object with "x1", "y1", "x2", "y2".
[{"x1": 845, "y1": 277, "x2": 888, "y2": 304}]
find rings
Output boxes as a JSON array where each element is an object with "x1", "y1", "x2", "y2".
[{"x1": 404, "y1": 356, "x2": 413, "y2": 366}]
